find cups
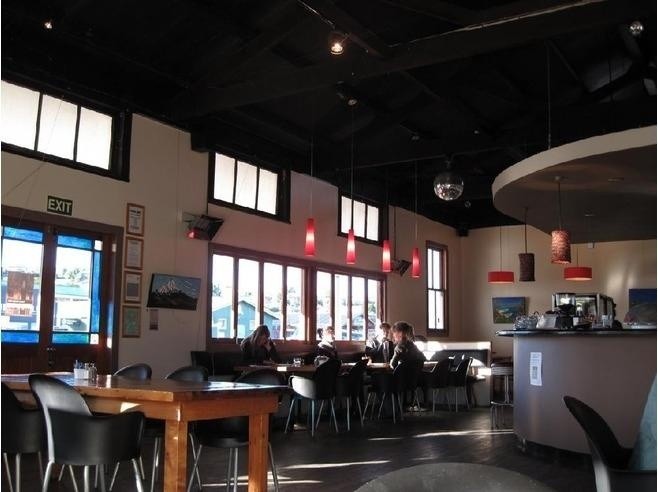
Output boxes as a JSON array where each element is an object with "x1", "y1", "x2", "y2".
[{"x1": 293, "y1": 358, "x2": 301, "y2": 367}]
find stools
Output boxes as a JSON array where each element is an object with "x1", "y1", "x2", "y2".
[{"x1": 491, "y1": 363, "x2": 513, "y2": 427}]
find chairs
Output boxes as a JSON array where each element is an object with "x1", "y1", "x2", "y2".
[
  {"x1": 284, "y1": 359, "x2": 344, "y2": 436},
  {"x1": 29, "y1": 374, "x2": 145, "y2": 492},
  {"x1": 187, "y1": 369, "x2": 286, "y2": 490},
  {"x1": 336, "y1": 357, "x2": 371, "y2": 432},
  {"x1": 363, "y1": 358, "x2": 425, "y2": 423},
  {"x1": 449, "y1": 357, "x2": 473, "y2": 410},
  {"x1": 109, "y1": 362, "x2": 153, "y2": 489},
  {"x1": 422, "y1": 355, "x2": 457, "y2": 415},
  {"x1": 563, "y1": 395, "x2": 658, "y2": 491},
  {"x1": 147, "y1": 365, "x2": 207, "y2": 492},
  {"x1": 2, "y1": 383, "x2": 46, "y2": 486}
]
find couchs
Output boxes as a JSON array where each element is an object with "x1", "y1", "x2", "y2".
[
  {"x1": 191, "y1": 351, "x2": 365, "y2": 375},
  {"x1": 428, "y1": 341, "x2": 492, "y2": 407}
]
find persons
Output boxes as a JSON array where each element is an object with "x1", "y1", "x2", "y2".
[
  {"x1": 311, "y1": 325, "x2": 339, "y2": 366},
  {"x1": 390, "y1": 321, "x2": 427, "y2": 381},
  {"x1": 232, "y1": 324, "x2": 283, "y2": 384},
  {"x1": 364, "y1": 323, "x2": 397, "y2": 363}
]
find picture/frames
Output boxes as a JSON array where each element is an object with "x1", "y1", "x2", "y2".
[
  {"x1": 126, "y1": 203, "x2": 148, "y2": 235},
  {"x1": 146, "y1": 272, "x2": 201, "y2": 311},
  {"x1": 491, "y1": 295, "x2": 527, "y2": 324},
  {"x1": 124, "y1": 237, "x2": 145, "y2": 269},
  {"x1": 123, "y1": 273, "x2": 141, "y2": 304},
  {"x1": 122, "y1": 306, "x2": 143, "y2": 338}
]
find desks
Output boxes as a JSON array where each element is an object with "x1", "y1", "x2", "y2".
[{"x1": 494, "y1": 326, "x2": 657, "y2": 471}]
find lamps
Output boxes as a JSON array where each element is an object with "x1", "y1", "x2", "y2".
[
  {"x1": 345, "y1": 98, "x2": 356, "y2": 264},
  {"x1": 519, "y1": 207, "x2": 535, "y2": 280},
  {"x1": 411, "y1": 135, "x2": 420, "y2": 278},
  {"x1": 434, "y1": 173, "x2": 464, "y2": 200},
  {"x1": 551, "y1": 175, "x2": 570, "y2": 263},
  {"x1": 304, "y1": 158, "x2": 316, "y2": 255},
  {"x1": 563, "y1": 248, "x2": 591, "y2": 280},
  {"x1": 381, "y1": 177, "x2": 393, "y2": 271},
  {"x1": 487, "y1": 225, "x2": 513, "y2": 284}
]
